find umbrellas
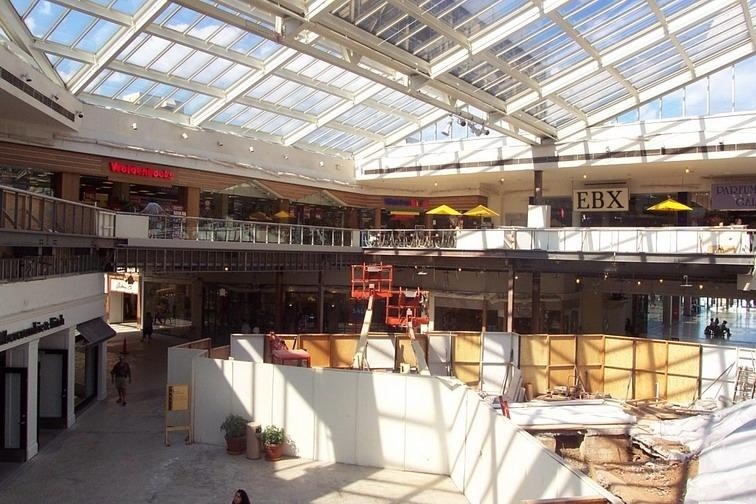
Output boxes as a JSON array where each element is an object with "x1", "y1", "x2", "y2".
[
  {"x1": 425, "y1": 205, "x2": 462, "y2": 229},
  {"x1": 463, "y1": 204, "x2": 500, "y2": 228},
  {"x1": 647, "y1": 198, "x2": 693, "y2": 226}
]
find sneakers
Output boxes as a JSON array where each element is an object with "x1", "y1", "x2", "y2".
[{"x1": 116, "y1": 397, "x2": 126, "y2": 407}]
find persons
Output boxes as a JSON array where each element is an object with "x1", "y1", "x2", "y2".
[
  {"x1": 231, "y1": 489, "x2": 250, "y2": 504},
  {"x1": 141, "y1": 197, "x2": 170, "y2": 229},
  {"x1": 110, "y1": 355, "x2": 131, "y2": 406},
  {"x1": 705, "y1": 318, "x2": 731, "y2": 336},
  {"x1": 240, "y1": 318, "x2": 252, "y2": 333},
  {"x1": 140, "y1": 311, "x2": 154, "y2": 344}
]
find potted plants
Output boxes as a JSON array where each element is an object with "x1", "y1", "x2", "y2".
[
  {"x1": 259, "y1": 424, "x2": 287, "y2": 461},
  {"x1": 219, "y1": 413, "x2": 250, "y2": 455}
]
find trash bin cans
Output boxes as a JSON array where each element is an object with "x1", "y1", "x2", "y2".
[{"x1": 245, "y1": 421, "x2": 261, "y2": 460}]
